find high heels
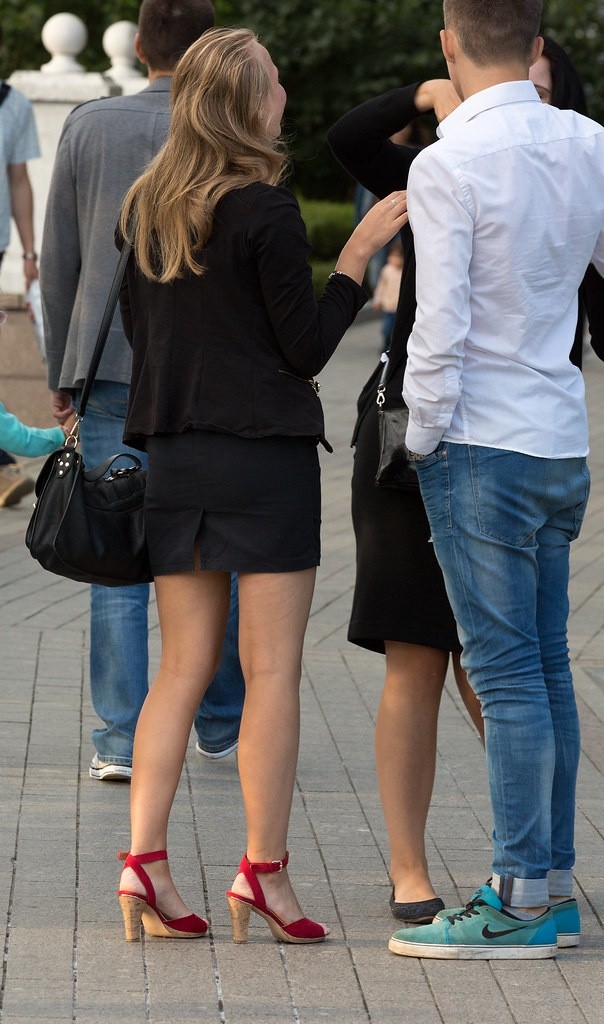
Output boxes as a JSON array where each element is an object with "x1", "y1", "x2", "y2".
[
  {"x1": 113, "y1": 850, "x2": 215, "y2": 944},
  {"x1": 221, "y1": 853, "x2": 330, "y2": 948}
]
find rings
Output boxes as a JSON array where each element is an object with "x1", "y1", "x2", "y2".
[{"x1": 391, "y1": 199, "x2": 396, "y2": 205}]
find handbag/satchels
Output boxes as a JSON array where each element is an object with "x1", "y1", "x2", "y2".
[
  {"x1": 22, "y1": 443, "x2": 167, "y2": 587},
  {"x1": 371, "y1": 398, "x2": 426, "y2": 522}
]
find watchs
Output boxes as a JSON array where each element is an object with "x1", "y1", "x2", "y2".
[{"x1": 21, "y1": 251, "x2": 40, "y2": 260}]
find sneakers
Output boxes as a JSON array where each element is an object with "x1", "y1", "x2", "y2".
[
  {"x1": 189, "y1": 732, "x2": 245, "y2": 765},
  {"x1": 434, "y1": 880, "x2": 583, "y2": 950},
  {"x1": 85, "y1": 747, "x2": 137, "y2": 780},
  {"x1": 386, "y1": 886, "x2": 565, "y2": 962},
  {"x1": 0, "y1": 465, "x2": 36, "y2": 513}
]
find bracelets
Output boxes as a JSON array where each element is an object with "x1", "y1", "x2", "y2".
[
  {"x1": 324, "y1": 269, "x2": 344, "y2": 279},
  {"x1": 58, "y1": 424, "x2": 72, "y2": 448}
]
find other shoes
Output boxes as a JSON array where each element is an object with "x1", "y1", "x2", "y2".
[{"x1": 386, "y1": 882, "x2": 445, "y2": 925}]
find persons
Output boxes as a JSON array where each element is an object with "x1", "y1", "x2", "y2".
[
  {"x1": 1, "y1": 64, "x2": 40, "y2": 509},
  {"x1": 326, "y1": 0, "x2": 600, "y2": 951},
  {"x1": 41, "y1": 4, "x2": 247, "y2": 781},
  {"x1": 0, "y1": 395, "x2": 79, "y2": 457},
  {"x1": 115, "y1": 26, "x2": 409, "y2": 944}
]
